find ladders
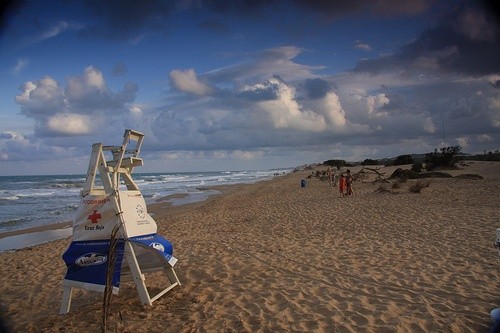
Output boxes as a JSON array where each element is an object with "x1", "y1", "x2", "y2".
[{"x1": 58, "y1": 129, "x2": 182, "y2": 316}]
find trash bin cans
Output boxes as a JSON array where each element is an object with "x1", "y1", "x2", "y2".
[{"x1": 300, "y1": 179, "x2": 306, "y2": 187}]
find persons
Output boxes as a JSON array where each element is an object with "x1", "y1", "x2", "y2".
[{"x1": 316, "y1": 162, "x2": 354, "y2": 197}]
